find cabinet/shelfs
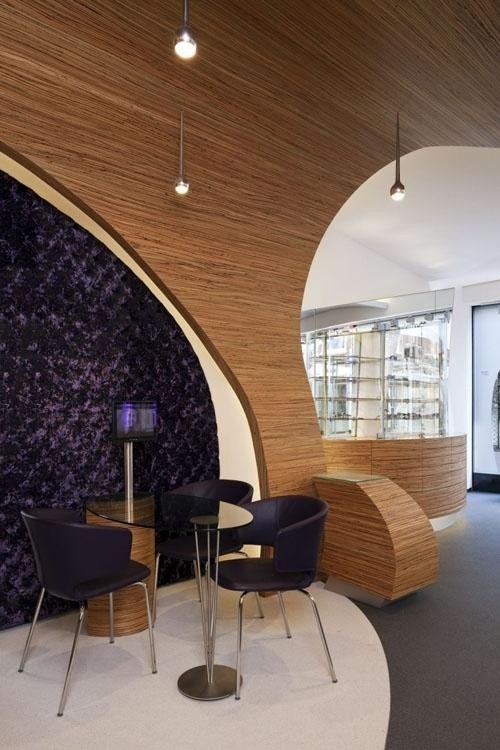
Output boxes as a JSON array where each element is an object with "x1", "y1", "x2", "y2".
[{"x1": 298, "y1": 285, "x2": 455, "y2": 438}]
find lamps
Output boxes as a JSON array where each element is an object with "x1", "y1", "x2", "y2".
[
  {"x1": 389, "y1": 112, "x2": 405, "y2": 201},
  {"x1": 173, "y1": 0, "x2": 197, "y2": 60},
  {"x1": 175, "y1": 111, "x2": 189, "y2": 195},
  {"x1": 112, "y1": 400, "x2": 159, "y2": 522}
]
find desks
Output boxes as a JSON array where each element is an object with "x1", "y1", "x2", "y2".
[{"x1": 84, "y1": 491, "x2": 254, "y2": 702}]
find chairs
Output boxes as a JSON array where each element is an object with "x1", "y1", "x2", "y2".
[
  {"x1": 205, "y1": 494, "x2": 338, "y2": 700},
  {"x1": 18, "y1": 507, "x2": 157, "y2": 716},
  {"x1": 151, "y1": 478, "x2": 264, "y2": 628}
]
